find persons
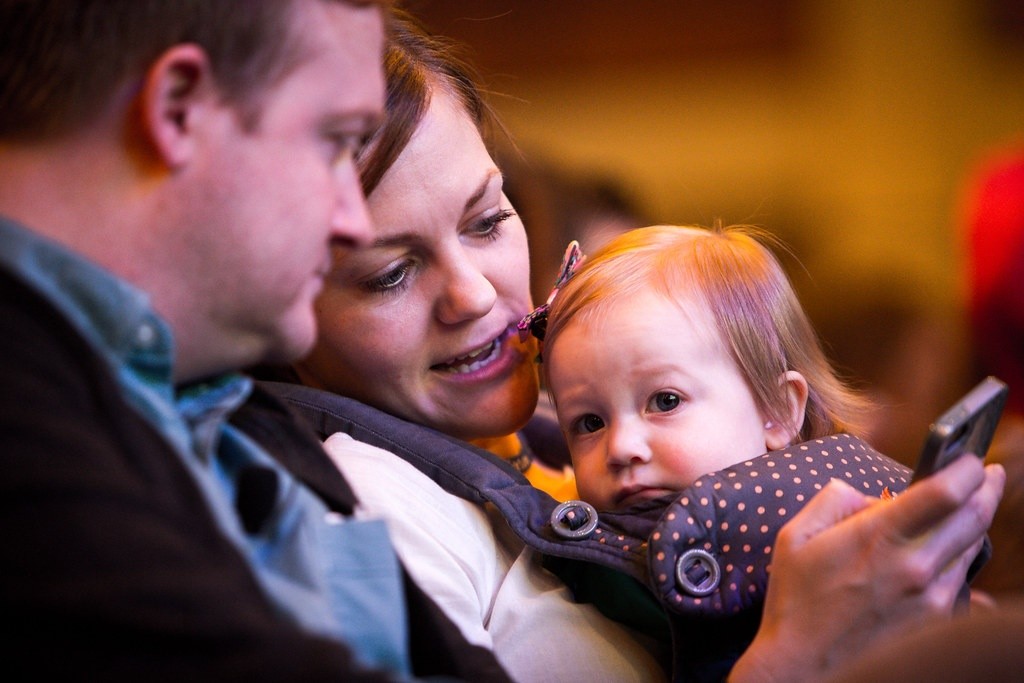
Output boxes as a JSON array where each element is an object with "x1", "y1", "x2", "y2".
[{"x1": 0, "y1": 0, "x2": 1024, "y2": 683}]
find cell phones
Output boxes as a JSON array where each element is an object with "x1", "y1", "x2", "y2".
[{"x1": 910, "y1": 377, "x2": 1009, "y2": 488}]
